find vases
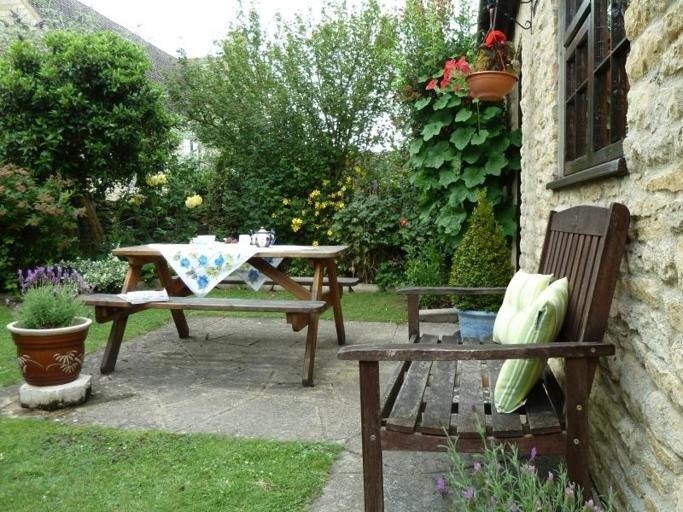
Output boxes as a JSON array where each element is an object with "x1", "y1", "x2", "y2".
[{"x1": 464, "y1": 70, "x2": 520, "y2": 102}]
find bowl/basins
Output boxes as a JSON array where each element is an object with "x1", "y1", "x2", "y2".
[{"x1": 198, "y1": 235, "x2": 216, "y2": 242}]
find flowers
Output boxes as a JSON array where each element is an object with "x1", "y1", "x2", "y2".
[{"x1": 475, "y1": 30, "x2": 518, "y2": 73}]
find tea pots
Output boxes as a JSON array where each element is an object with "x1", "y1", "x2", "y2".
[{"x1": 250, "y1": 227, "x2": 276, "y2": 247}]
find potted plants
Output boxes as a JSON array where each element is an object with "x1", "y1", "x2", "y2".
[
  {"x1": 444, "y1": 185, "x2": 513, "y2": 339},
  {"x1": 7, "y1": 285, "x2": 94, "y2": 385}
]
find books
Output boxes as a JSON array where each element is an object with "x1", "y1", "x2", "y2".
[{"x1": 116, "y1": 288, "x2": 170, "y2": 305}]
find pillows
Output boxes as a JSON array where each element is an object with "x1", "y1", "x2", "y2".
[
  {"x1": 491, "y1": 267, "x2": 552, "y2": 343},
  {"x1": 491, "y1": 277, "x2": 570, "y2": 409}
]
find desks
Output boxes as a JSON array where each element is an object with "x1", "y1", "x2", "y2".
[{"x1": 98, "y1": 244, "x2": 351, "y2": 387}]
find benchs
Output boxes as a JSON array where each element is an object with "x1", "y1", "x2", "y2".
[
  {"x1": 82, "y1": 291, "x2": 326, "y2": 330},
  {"x1": 171, "y1": 276, "x2": 360, "y2": 293},
  {"x1": 342, "y1": 201, "x2": 632, "y2": 512}
]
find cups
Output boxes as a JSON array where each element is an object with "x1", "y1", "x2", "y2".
[{"x1": 238, "y1": 235, "x2": 251, "y2": 244}]
